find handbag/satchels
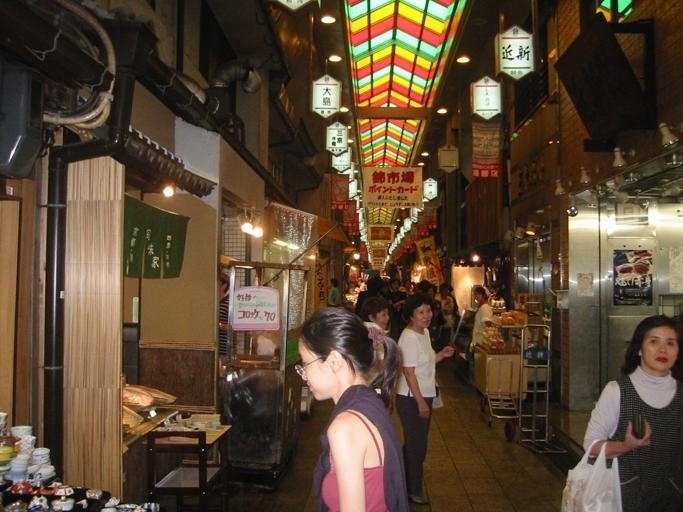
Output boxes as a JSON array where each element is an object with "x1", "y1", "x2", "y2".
[
  {"x1": 561, "y1": 440, "x2": 625, "y2": 512},
  {"x1": 434, "y1": 383, "x2": 445, "y2": 409}
]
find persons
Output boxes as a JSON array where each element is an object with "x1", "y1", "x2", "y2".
[
  {"x1": 583, "y1": 314, "x2": 683, "y2": 512},
  {"x1": 299, "y1": 306, "x2": 409, "y2": 512},
  {"x1": 328, "y1": 276, "x2": 494, "y2": 505},
  {"x1": 217, "y1": 271, "x2": 238, "y2": 363}
]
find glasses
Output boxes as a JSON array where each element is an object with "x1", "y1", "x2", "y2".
[{"x1": 294, "y1": 356, "x2": 326, "y2": 375}]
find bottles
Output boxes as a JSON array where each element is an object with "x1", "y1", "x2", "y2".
[{"x1": 481, "y1": 326, "x2": 504, "y2": 352}]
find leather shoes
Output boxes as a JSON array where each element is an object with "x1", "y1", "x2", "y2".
[{"x1": 409, "y1": 494, "x2": 427, "y2": 505}]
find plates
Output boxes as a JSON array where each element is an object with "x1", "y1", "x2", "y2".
[{"x1": 616, "y1": 259, "x2": 652, "y2": 278}]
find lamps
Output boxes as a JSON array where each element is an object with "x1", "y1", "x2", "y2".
[
  {"x1": 236, "y1": 213, "x2": 253, "y2": 232},
  {"x1": 514, "y1": 227, "x2": 525, "y2": 240},
  {"x1": 612, "y1": 146, "x2": 634, "y2": 169},
  {"x1": 578, "y1": 164, "x2": 592, "y2": 189},
  {"x1": 656, "y1": 123, "x2": 680, "y2": 151},
  {"x1": 554, "y1": 177, "x2": 573, "y2": 197},
  {"x1": 504, "y1": 229, "x2": 512, "y2": 243},
  {"x1": 246, "y1": 207, "x2": 263, "y2": 242},
  {"x1": 567, "y1": 170, "x2": 632, "y2": 216},
  {"x1": 527, "y1": 222, "x2": 544, "y2": 237}
]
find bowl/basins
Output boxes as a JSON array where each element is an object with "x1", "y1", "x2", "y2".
[
  {"x1": 12, "y1": 425, "x2": 50, "y2": 471},
  {"x1": 0, "y1": 436, "x2": 21, "y2": 467}
]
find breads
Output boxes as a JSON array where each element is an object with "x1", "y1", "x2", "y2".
[{"x1": 492, "y1": 311, "x2": 525, "y2": 325}]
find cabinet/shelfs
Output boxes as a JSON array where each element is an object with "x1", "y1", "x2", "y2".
[
  {"x1": 473, "y1": 311, "x2": 528, "y2": 400},
  {"x1": 147, "y1": 416, "x2": 233, "y2": 512}
]
